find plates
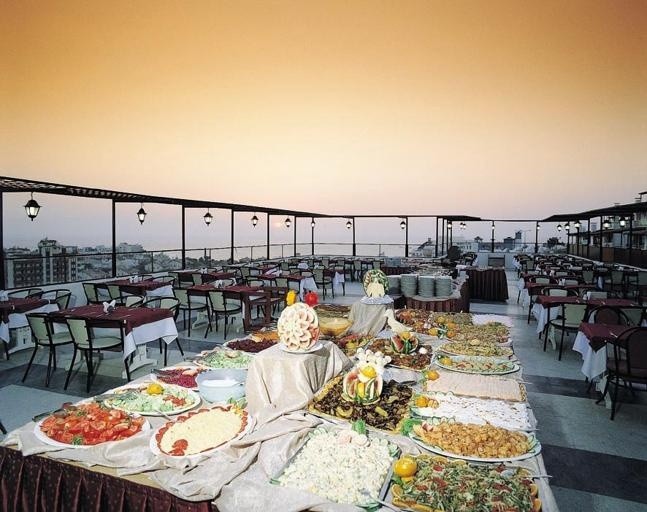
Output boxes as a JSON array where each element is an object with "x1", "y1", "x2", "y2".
[
  {"x1": 106, "y1": 383, "x2": 200, "y2": 415},
  {"x1": 382, "y1": 273, "x2": 453, "y2": 299},
  {"x1": 361, "y1": 310, "x2": 523, "y2": 376},
  {"x1": 151, "y1": 404, "x2": 254, "y2": 462},
  {"x1": 408, "y1": 418, "x2": 542, "y2": 461},
  {"x1": 34, "y1": 406, "x2": 146, "y2": 448},
  {"x1": 221, "y1": 328, "x2": 276, "y2": 357},
  {"x1": 193, "y1": 351, "x2": 255, "y2": 371},
  {"x1": 151, "y1": 364, "x2": 212, "y2": 392}
]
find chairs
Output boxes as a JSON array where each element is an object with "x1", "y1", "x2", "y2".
[
  {"x1": 289, "y1": 254, "x2": 506, "y2": 284},
  {"x1": 512, "y1": 254, "x2": 646, "y2": 420},
  {"x1": 2, "y1": 260, "x2": 305, "y2": 393}
]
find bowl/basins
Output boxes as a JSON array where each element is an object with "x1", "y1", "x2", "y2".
[
  {"x1": 193, "y1": 369, "x2": 248, "y2": 403},
  {"x1": 318, "y1": 316, "x2": 354, "y2": 337}
]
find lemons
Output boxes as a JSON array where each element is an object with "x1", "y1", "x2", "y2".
[
  {"x1": 426, "y1": 370, "x2": 440, "y2": 381},
  {"x1": 414, "y1": 395, "x2": 430, "y2": 408},
  {"x1": 146, "y1": 383, "x2": 165, "y2": 397},
  {"x1": 427, "y1": 328, "x2": 437, "y2": 337},
  {"x1": 439, "y1": 356, "x2": 453, "y2": 367},
  {"x1": 391, "y1": 451, "x2": 542, "y2": 512},
  {"x1": 356, "y1": 367, "x2": 376, "y2": 397},
  {"x1": 345, "y1": 342, "x2": 356, "y2": 350}
]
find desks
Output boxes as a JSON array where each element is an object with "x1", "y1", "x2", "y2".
[{"x1": 381, "y1": 261, "x2": 509, "y2": 313}]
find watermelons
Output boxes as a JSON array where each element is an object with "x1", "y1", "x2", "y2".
[{"x1": 390, "y1": 334, "x2": 420, "y2": 355}]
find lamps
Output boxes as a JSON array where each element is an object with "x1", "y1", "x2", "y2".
[
  {"x1": 311, "y1": 221, "x2": 316, "y2": 227},
  {"x1": 536, "y1": 224, "x2": 541, "y2": 230},
  {"x1": 400, "y1": 221, "x2": 406, "y2": 229},
  {"x1": 602, "y1": 217, "x2": 626, "y2": 231},
  {"x1": 448, "y1": 222, "x2": 466, "y2": 230},
  {"x1": 284, "y1": 219, "x2": 292, "y2": 227},
  {"x1": 556, "y1": 222, "x2": 582, "y2": 232},
  {"x1": 346, "y1": 221, "x2": 351, "y2": 229},
  {"x1": 203, "y1": 213, "x2": 213, "y2": 226},
  {"x1": 23, "y1": 191, "x2": 42, "y2": 222},
  {"x1": 137, "y1": 208, "x2": 148, "y2": 225},
  {"x1": 251, "y1": 216, "x2": 259, "y2": 226}
]
